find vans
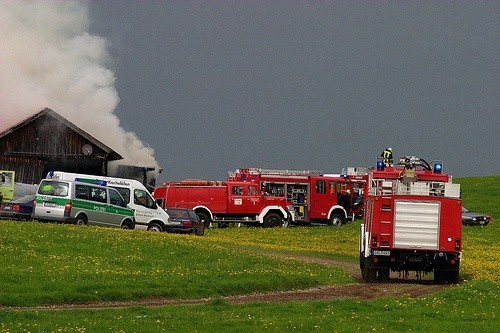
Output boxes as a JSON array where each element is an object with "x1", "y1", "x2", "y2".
[
  {"x1": 0, "y1": 171, "x2": 172, "y2": 233},
  {"x1": 34, "y1": 179, "x2": 135, "y2": 230}
]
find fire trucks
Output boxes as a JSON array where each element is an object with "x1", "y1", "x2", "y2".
[
  {"x1": 152, "y1": 179, "x2": 288, "y2": 228},
  {"x1": 359, "y1": 147, "x2": 461, "y2": 283},
  {"x1": 228, "y1": 166, "x2": 369, "y2": 220}
]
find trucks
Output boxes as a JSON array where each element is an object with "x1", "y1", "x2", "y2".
[{"x1": 227, "y1": 173, "x2": 354, "y2": 227}]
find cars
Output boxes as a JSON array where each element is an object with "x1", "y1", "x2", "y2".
[
  {"x1": 462, "y1": 206, "x2": 492, "y2": 228},
  {"x1": 164, "y1": 208, "x2": 204, "y2": 236},
  {"x1": 0, "y1": 194, "x2": 35, "y2": 223}
]
